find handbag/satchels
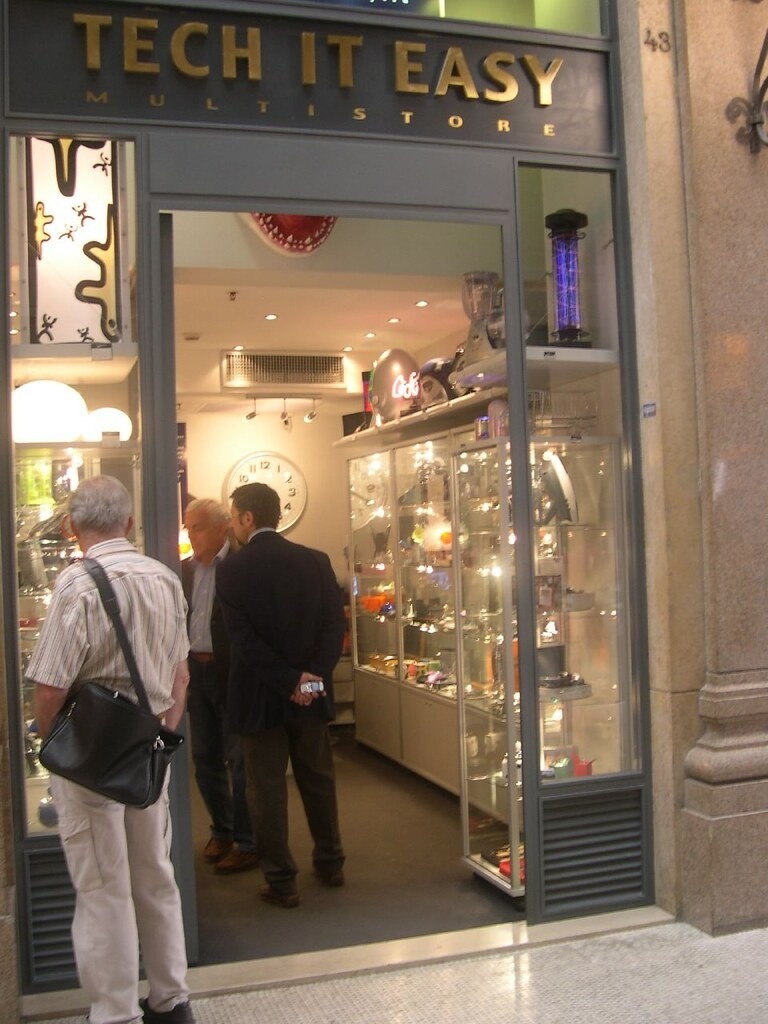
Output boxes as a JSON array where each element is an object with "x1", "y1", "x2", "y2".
[{"x1": 38, "y1": 680, "x2": 185, "y2": 811}]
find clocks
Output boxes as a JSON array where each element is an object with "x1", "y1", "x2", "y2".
[{"x1": 223, "y1": 451, "x2": 308, "y2": 536}]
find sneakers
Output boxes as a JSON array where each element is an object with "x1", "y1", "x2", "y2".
[
  {"x1": 213, "y1": 848, "x2": 249, "y2": 875},
  {"x1": 311, "y1": 862, "x2": 344, "y2": 888},
  {"x1": 139, "y1": 998, "x2": 196, "y2": 1024},
  {"x1": 202, "y1": 837, "x2": 234, "y2": 862},
  {"x1": 258, "y1": 883, "x2": 299, "y2": 908}
]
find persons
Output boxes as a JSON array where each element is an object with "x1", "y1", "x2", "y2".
[
  {"x1": 181, "y1": 497, "x2": 257, "y2": 870},
  {"x1": 210, "y1": 483, "x2": 344, "y2": 901},
  {"x1": 25, "y1": 475, "x2": 197, "y2": 1024}
]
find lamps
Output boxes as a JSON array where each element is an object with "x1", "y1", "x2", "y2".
[
  {"x1": 245, "y1": 398, "x2": 258, "y2": 419},
  {"x1": 305, "y1": 399, "x2": 318, "y2": 423},
  {"x1": 281, "y1": 398, "x2": 288, "y2": 420}
]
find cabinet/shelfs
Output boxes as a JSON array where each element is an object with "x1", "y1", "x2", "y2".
[
  {"x1": 333, "y1": 387, "x2": 638, "y2": 899},
  {"x1": 17, "y1": 536, "x2": 87, "y2": 786}
]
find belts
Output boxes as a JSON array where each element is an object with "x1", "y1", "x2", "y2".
[{"x1": 189, "y1": 652, "x2": 214, "y2": 663}]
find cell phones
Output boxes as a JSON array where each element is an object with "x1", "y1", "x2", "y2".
[{"x1": 301, "y1": 681, "x2": 324, "y2": 693}]
